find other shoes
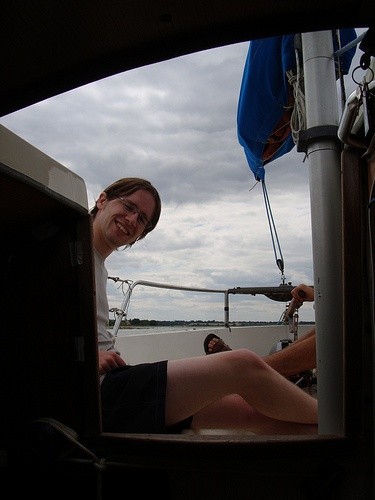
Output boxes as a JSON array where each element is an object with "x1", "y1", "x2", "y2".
[{"x1": 203, "y1": 333, "x2": 232, "y2": 355}]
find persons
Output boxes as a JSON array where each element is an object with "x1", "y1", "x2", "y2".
[
  {"x1": 203, "y1": 283, "x2": 318, "y2": 380},
  {"x1": 88, "y1": 176, "x2": 319, "y2": 435}
]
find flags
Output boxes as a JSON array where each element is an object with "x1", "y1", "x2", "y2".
[{"x1": 237, "y1": 23, "x2": 359, "y2": 183}]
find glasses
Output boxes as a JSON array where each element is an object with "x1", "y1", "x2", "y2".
[{"x1": 118, "y1": 196, "x2": 153, "y2": 229}]
